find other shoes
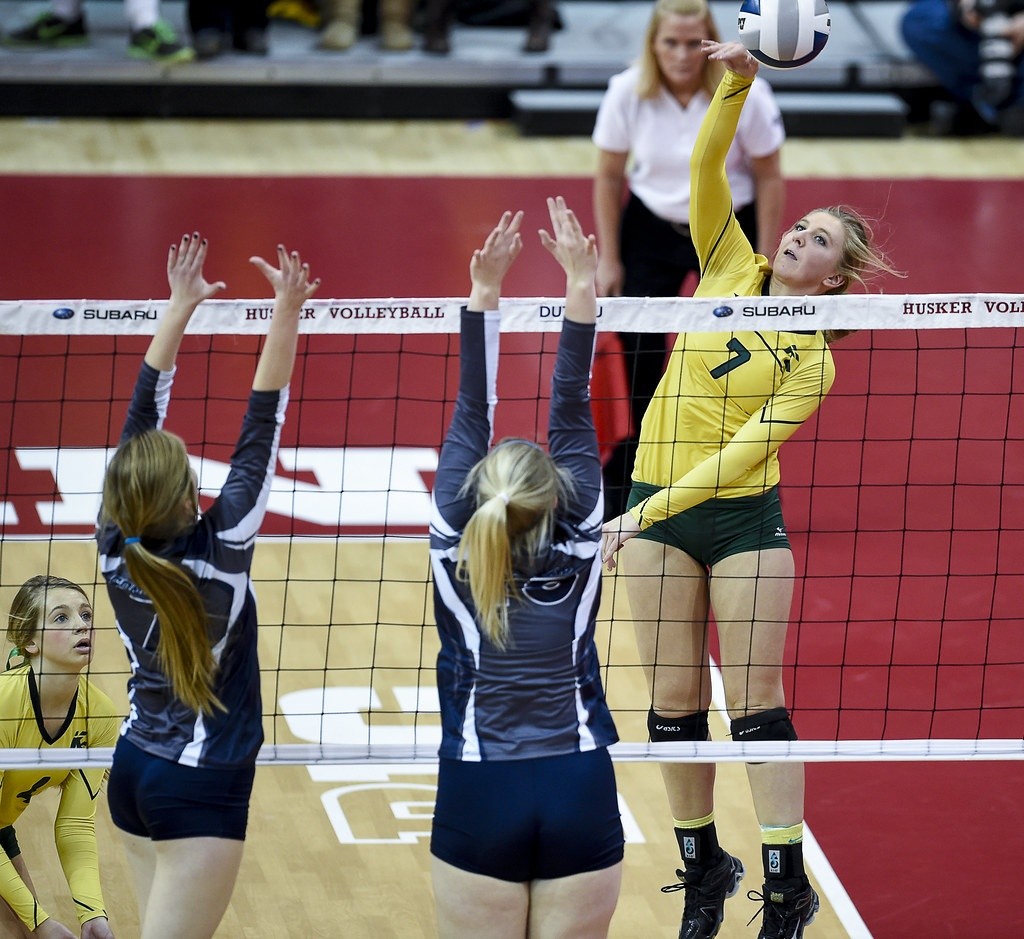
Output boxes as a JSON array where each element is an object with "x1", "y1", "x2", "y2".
[
  {"x1": 524, "y1": 20, "x2": 551, "y2": 53},
  {"x1": 424, "y1": 15, "x2": 452, "y2": 52},
  {"x1": 190, "y1": 26, "x2": 270, "y2": 60},
  {"x1": 379, "y1": 16, "x2": 415, "y2": 51},
  {"x1": 316, "y1": 12, "x2": 359, "y2": 52}
]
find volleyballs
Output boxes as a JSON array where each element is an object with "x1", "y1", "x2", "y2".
[{"x1": 737, "y1": 0, "x2": 832, "y2": 71}]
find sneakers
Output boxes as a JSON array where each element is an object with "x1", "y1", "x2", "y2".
[
  {"x1": 0, "y1": 11, "x2": 92, "y2": 51},
  {"x1": 659, "y1": 847, "x2": 745, "y2": 939},
  {"x1": 127, "y1": 15, "x2": 197, "y2": 66},
  {"x1": 745, "y1": 872, "x2": 820, "y2": 939}
]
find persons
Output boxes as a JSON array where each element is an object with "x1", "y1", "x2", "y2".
[
  {"x1": 0, "y1": 575, "x2": 122, "y2": 939},
  {"x1": 587, "y1": 0, "x2": 787, "y2": 436},
  {"x1": 0, "y1": 0, "x2": 568, "y2": 67},
  {"x1": 93, "y1": 232, "x2": 321, "y2": 939},
  {"x1": 600, "y1": 41, "x2": 908, "y2": 939},
  {"x1": 426, "y1": 196, "x2": 628, "y2": 939}
]
